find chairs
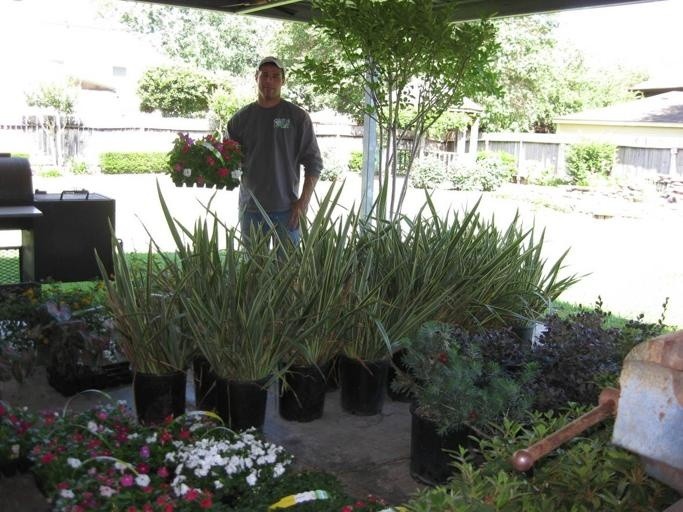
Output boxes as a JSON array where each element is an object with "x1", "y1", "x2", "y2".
[{"x1": 0, "y1": 157, "x2": 43, "y2": 282}]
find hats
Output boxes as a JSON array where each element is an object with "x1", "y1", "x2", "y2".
[{"x1": 258, "y1": 57, "x2": 284, "y2": 73}]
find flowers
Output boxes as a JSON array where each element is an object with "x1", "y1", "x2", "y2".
[{"x1": 162, "y1": 131, "x2": 244, "y2": 187}]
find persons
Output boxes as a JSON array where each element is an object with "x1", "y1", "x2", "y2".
[{"x1": 225, "y1": 54, "x2": 323, "y2": 266}]
